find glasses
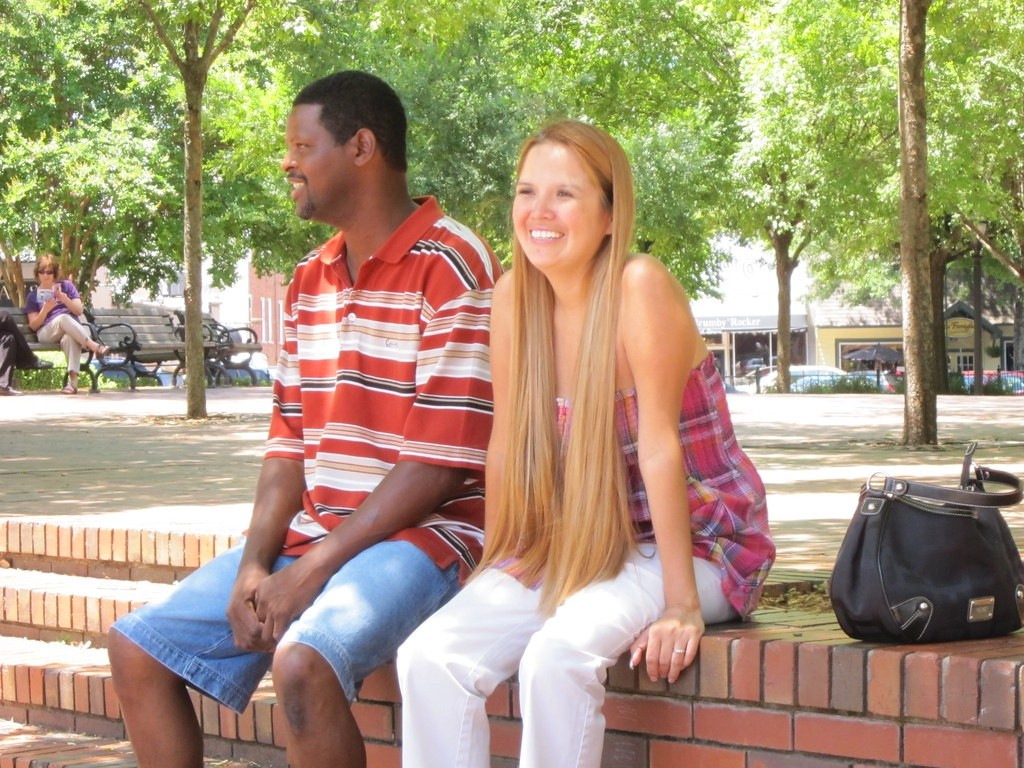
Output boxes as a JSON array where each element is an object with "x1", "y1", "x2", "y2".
[{"x1": 38, "y1": 270, "x2": 54, "y2": 274}]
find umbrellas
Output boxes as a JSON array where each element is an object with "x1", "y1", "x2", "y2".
[{"x1": 842, "y1": 342, "x2": 904, "y2": 388}]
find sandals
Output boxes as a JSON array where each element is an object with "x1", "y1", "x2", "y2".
[
  {"x1": 60, "y1": 383, "x2": 77, "y2": 394},
  {"x1": 96, "y1": 344, "x2": 110, "y2": 360}
]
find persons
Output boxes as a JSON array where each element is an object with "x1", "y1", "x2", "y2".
[
  {"x1": 396, "y1": 121, "x2": 776, "y2": 768},
  {"x1": 107, "y1": 70, "x2": 504, "y2": 768},
  {"x1": 25, "y1": 252, "x2": 111, "y2": 394},
  {"x1": 0, "y1": 309, "x2": 54, "y2": 396}
]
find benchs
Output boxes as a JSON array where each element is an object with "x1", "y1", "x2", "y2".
[
  {"x1": 1, "y1": 306, "x2": 100, "y2": 393},
  {"x1": 173, "y1": 309, "x2": 263, "y2": 387},
  {"x1": 82, "y1": 306, "x2": 217, "y2": 394}
]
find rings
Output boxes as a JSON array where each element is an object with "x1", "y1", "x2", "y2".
[{"x1": 673, "y1": 648, "x2": 685, "y2": 653}]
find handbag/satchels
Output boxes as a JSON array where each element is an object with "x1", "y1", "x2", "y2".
[{"x1": 829, "y1": 443, "x2": 1024, "y2": 644}]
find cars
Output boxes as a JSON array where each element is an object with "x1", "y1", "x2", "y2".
[{"x1": 713, "y1": 365, "x2": 1024, "y2": 396}]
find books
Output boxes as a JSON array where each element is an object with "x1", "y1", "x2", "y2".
[{"x1": 37, "y1": 283, "x2": 62, "y2": 303}]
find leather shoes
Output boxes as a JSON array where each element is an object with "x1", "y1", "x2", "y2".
[
  {"x1": 0, "y1": 386, "x2": 22, "y2": 396},
  {"x1": 22, "y1": 357, "x2": 54, "y2": 370}
]
